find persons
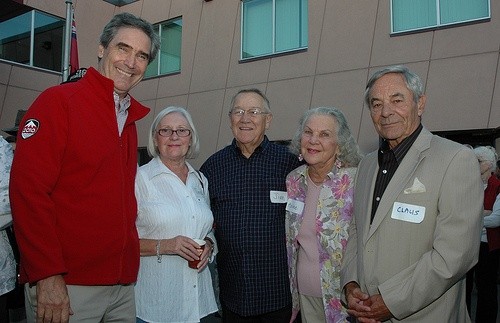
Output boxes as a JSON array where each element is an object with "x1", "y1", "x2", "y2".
[
  {"x1": 466, "y1": 146, "x2": 500, "y2": 323},
  {"x1": 0, "y1": 134, "x2": 17, "y2": 323},
  {"x1": 9, "y1": 13, "x2": 161, "y2": 323},
  {"x1": 199, "y1": 88, "x2": 307, "y2": 323},
  {"x1": 134, "y1": 106, "x2": 222, "y2": 323},
  {"x1": 340, "y1": 65, "x2": 483, "y2": 323},
  {"x1": 285, "y1": 107, "x2": 366, "y2": 323}
]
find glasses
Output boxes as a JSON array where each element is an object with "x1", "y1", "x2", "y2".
[
  {"x1": 156, "y1": 128, "x2": 191, "y2": 137},
  {"x1": 229, "y1": 108, "x2": 269, "y2": 118},
  {"x1": 478, "y1": 159, "x2": 490, "y2": 164}
]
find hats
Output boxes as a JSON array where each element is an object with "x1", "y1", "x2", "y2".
[{"x1": 1, "y1": 109, "x2": 30, "y2": 136}]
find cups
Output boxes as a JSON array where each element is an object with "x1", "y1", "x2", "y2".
[{"x1": 188, "y1": 239, "x2": 206, "y2": 269}]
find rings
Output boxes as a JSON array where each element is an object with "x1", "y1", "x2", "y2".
[{"x1": 206, "y1": 257, "x2": 210, "y2": 261}]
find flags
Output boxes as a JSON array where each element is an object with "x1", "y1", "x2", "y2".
[{"x1": 69, "y1": 14, "x2": 79, "y2": 75}]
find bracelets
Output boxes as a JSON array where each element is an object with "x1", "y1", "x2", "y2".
[
  {"x1": 157, "y1": 239, "x2": 162, "y2": 263},
  {"x1": 205, "y1": 237, "x2": 215, "y2": 254}
]
